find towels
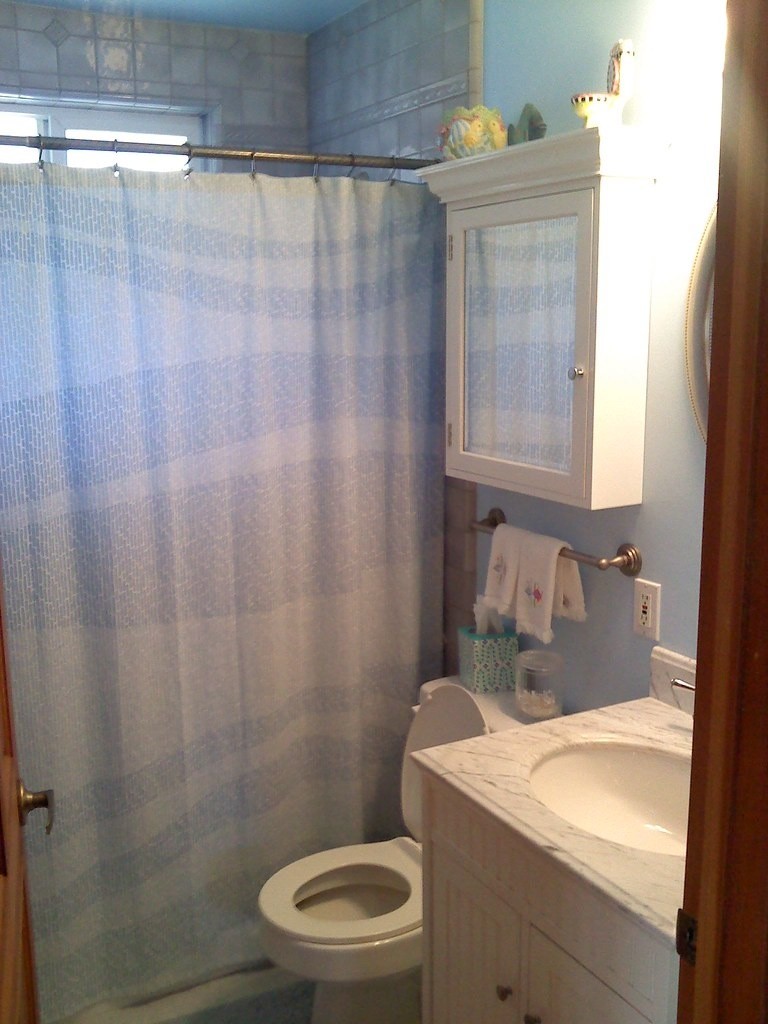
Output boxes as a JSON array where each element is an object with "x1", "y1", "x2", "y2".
[{"x1": 485, "y1": 522, "x2": 588, "y2": 645}]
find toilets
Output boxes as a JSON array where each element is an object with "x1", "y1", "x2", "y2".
[{"x1": 255, "y1": 670, "x2": 569, "y2": 1024}]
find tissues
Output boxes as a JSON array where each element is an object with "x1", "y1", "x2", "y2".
[{"x1": 457, "y1": 592, "x2": 520, "y2": 695}]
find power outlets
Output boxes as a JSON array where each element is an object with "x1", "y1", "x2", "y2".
[{"x1": 633, "y1": 578, "x2": 661, "y2": 641}]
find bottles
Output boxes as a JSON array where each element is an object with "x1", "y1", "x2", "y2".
[{"x1": 513, "y1": 648, "x2": 566, "y2": 719}]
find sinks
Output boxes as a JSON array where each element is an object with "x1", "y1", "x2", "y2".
[{"x1": 527, "y1": 738, "x2": 696, "y2": 858}]
